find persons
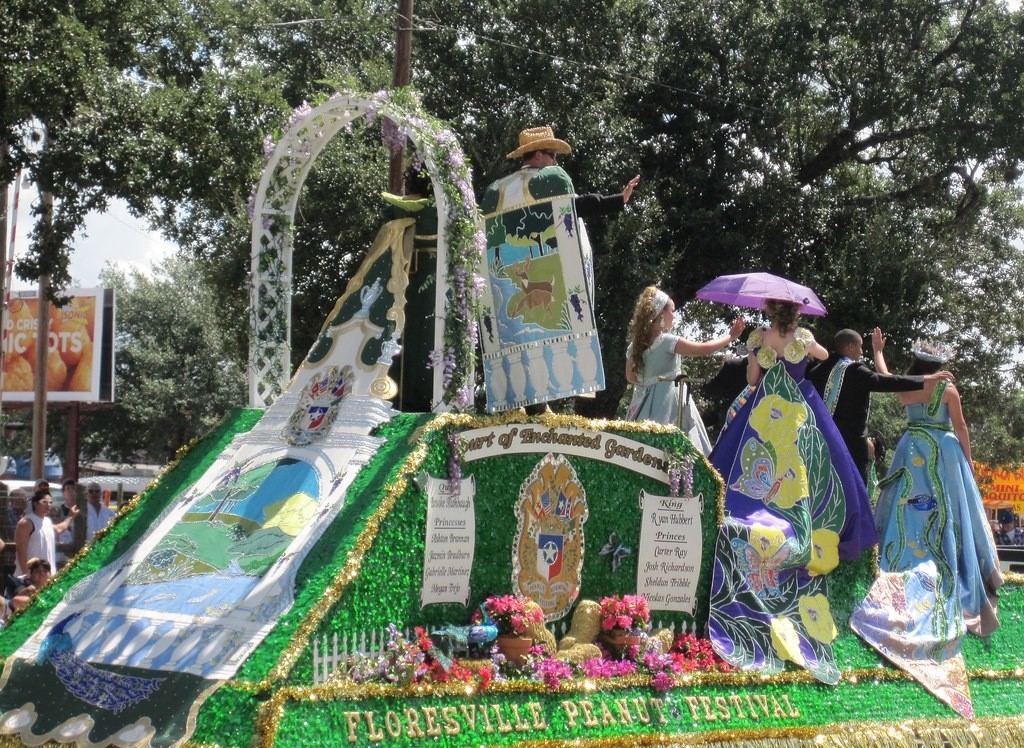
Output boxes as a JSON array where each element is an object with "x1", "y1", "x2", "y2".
[
  {"x1": 1, "y1": 480, "x2": 129, "y2": 626},
  {"x1": 626, "y1": 286, "x2": 746, "y2": 457},
  {"x1": 993, "y1": 508, "x2": 1023, "y2": 573},
  {"x1": 871, "y1": 329, "x2": 1003, "y2": 589},
  {"x1": 708, "y1": 297, "x2": 881, "y2": 562},
  {"x1": 805, "y1": 327, "x2": 954, "y2": 476},
  {"x1": 481, "y1": 125, "x2": 642, "y2": 415}
]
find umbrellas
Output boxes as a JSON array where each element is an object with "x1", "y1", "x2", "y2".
[{"x1": 694, "y1": 272, "x2": 828, "y2": 339}]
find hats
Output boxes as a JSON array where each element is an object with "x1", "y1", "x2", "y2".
[
  {"x1": 998, "y1": 511, "x2": 1014, "y2": 523},
  {"x1": 63, "y1": 477, "x2": 75, "y2": 486},
  {"x1": 506, "y1": 127, "x2": 571, "y2": 159},
  {"x1": 33, "y1": 479, "x2": 49, "y2": 488},
  {"x1": 914, "y1": 341, "x2": 958, "y2": 364}
]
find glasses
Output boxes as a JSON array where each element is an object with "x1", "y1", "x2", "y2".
[
  {"x1": 88, "y1": 489, "x2": 101, "y2": 493},
  {"x1": 16, "y1": 498, "x2": 28, "y2": 502},
  {"x1": 541, "y1": 150, "x2": 556, "y2": 160},
  {"x1": 34, "y1": 499, "x2": 53, "y2": 506}
]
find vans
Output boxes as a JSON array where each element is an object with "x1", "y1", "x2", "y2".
[{"x1": 0, "y1": 458, "x2": 167, "y2": 571}]
